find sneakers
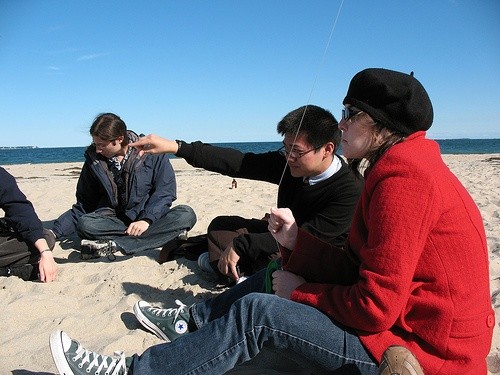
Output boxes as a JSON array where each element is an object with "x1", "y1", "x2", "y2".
[
  {"x1": 81, "y1": 239, "x2": 118, "y2": 261},
  {"x1": 133, "y1": 300, "x2": 194, "y2": 343},
  {"x1": 178, "y1": 230, "x2": 187, "y2": 240},
  {"x1": 49, "y1": 330, "x2": 127, "y2": 375}
]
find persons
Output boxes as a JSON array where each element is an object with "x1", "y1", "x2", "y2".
[
  {"x1": 50, "y1": 68, "x2": 494, "y2": 375},
  {"x1": 0, "y1": 167, "x2": 58, "y2": 283},
  {"x1": 377, "y1": 345, "x2": 424, "y2": 375},
  {"x1": 127, "y1": 106, "x2": 365, "y2": 277},
  {"x1": 51, "y1": 113, "x2": 198, "y2": 261}
]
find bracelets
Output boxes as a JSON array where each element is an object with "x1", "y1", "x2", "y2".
[
  {"x1": 40, "y1": 248, "x2": 51, "y2": 253},
  {"x1": 176, "y1": 139, "x2": 181, "y2": 150}
]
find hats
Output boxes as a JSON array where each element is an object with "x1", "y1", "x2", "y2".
[{"x1": 343, "y1": 68, "x2": 433, "y2": 136}]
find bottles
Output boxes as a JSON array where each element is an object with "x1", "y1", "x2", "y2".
[{"x1": 232, "y1": 178, "x2": 237, "y2": 189}]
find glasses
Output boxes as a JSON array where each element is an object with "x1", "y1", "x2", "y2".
[
  {"x1": 278, "y1": 146, "x2": 320, "y2": 159},
  {"x1": 342, "y1": 108, "x2": 364, "y2": 120},
  {"x1": 91, "y1": 136, "x2": 121, "y2": 148}
]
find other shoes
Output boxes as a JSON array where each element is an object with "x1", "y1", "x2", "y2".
[
  {"x1": 10, "y1": 263, "x2": 39, "y2": 282},
  {"x1": 198, "y1": 252, "x2": 214, "y2": 272},
  {"x1": 376, "y1": 345, "x2": 425, "y2": 375}
]
